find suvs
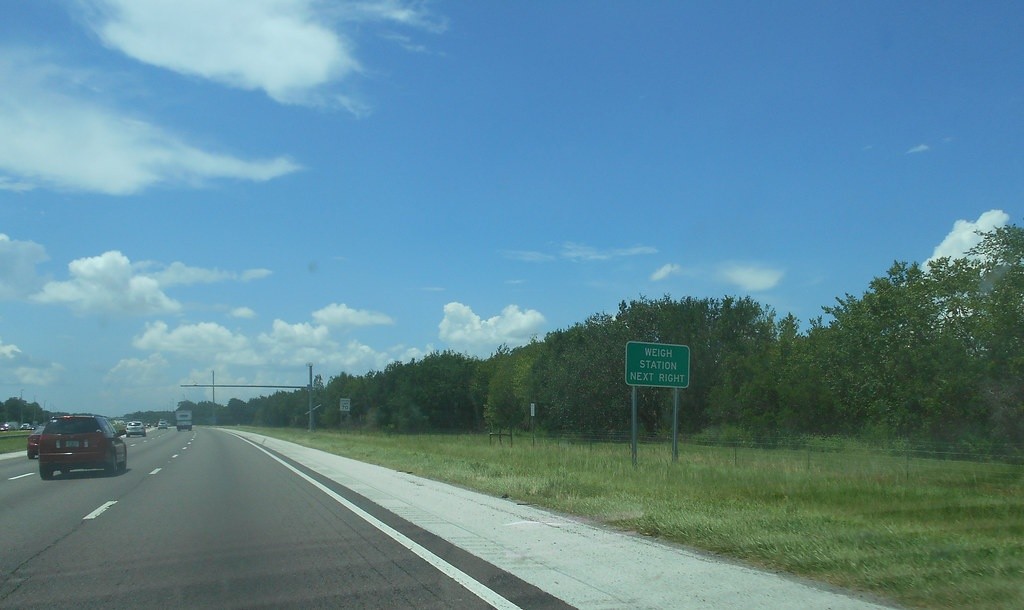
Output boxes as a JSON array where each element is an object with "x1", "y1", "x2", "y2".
[
  {"x1": 38, "y1": 413, "x2": 127, "y2": 480},
  {"x1": 20, "y1": 424, "x2": 30, "y2": 430},
  {"x1": 158, "y1": 421, "x2": 168, "y2": 429},
  {"x1": 27, "y1": 426, "x2": 45, "y2": 459},
  {"x1": 125, "y1": 421, "x2": 147, "y2": 437}
]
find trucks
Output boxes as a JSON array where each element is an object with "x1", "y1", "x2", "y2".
[{"x1": 176, "y1": 410, "x2": 193, "y2": 431}]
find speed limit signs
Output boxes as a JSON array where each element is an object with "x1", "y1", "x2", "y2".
[{"x1": 340, "y1": 398, "x2": 350, "y2": 412}]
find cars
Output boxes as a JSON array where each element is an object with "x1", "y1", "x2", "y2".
[
  {"x1": 146, "y1": 424, "x2": 151, "y2": 428},
  {"x1": 32, "y1": 426, "x2": 37, "y2": 430}
]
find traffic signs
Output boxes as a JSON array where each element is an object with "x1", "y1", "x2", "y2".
[{"x1": 625, "y1": 342, "x2": 690, "y2": 388}]
